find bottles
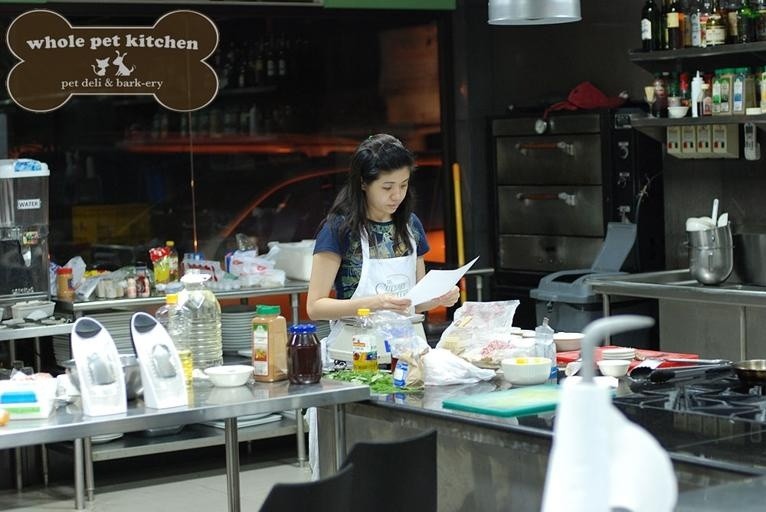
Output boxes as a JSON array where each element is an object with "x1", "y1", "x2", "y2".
[
  {"x1": 166, "y1": 240, "x2": 178, "y2": 280},
  {"x1": 56, "y1": 266, "x2": 75, "y2": 297},
  {"x1": 706, "y1": 0, "x2": 726, "y2": 45},
  {"x1": 252, "y1": 305, "x2": 287, "y2": 383},
  {"x1": 141, "y1": 275, "x2": 151, "y2": 297},
  {"x1": 666, "y1": 0, "x2": 683, "y2": 50},
  {"x1": 737, "y1": 0, "x2": 754, "y2": 43},
  {"x1": 352, "y1": 309, "x2": 379, "y2": 372},
  {"x1": 159, "y1": 294, "x2": 192, "y2": 389},
  {"x1": 136, "y1": 273, "x2": 142, "y2": 298},
  {"x1": 640, "y1": 1, "x2": 659, "y2": 48},
  {"x1": 127, "y1": 275, "x2": 136, "y2": 297}
]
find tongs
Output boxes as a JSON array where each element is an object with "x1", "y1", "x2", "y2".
[{"x1": 631, "y1": 363, "x2": 731, "y2": 382}]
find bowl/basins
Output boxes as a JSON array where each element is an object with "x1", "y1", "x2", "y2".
[
  {"x1": 602, "y1": 349, "x2": 636, "y2": 361},
  {"x1": 204, "y1": 364, "x2": 255, "y2": 387},
  {"x1": 597, "y1": 359, "x2": 631, "y2": 376},
  {"x1": 501, "y1": 357, "x2": 551, "y2": 385},
  {"x1": 732, "y1": 358, "x2": 766, "y2": 385},
  {"x1": 551, "y1": 333, "x2": 586, "y2": 352},
  {"x1": 65, "y1": 353, "x2": 146, "y2": 400}
]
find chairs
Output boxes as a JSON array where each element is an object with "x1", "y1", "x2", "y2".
[
  {"x1": 258, "y1": 469, "x2": 381, "y2": 512},
  {"x1": 339, "y1": 425, "x2": 437, "y2": 512}
]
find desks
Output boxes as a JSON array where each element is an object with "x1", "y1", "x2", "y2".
[
  {"x1": 0, "y1": 375, "x2": 371, "y2": 511},
  {"x1": 0, "y1": 318, "x2": 75, "y2": 493}
]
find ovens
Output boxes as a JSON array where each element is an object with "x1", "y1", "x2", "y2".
[{"x1": 487, "y1": 102, "x2": 664, "y2": 291}]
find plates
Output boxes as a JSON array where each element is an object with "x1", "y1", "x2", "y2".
[
  {"x1": 217, "y1": 304, "x2": 257, "y2": 356},
  {"x1": 52, "y1": 308, "x2": 140, "y2": 367},
  {"x1": 286, "y1": 320, "x2": 330, "y2": 342}
]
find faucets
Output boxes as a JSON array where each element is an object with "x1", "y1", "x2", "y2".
[{"x1": 581, "y1": 316, "x2": 657, "y2": 381}]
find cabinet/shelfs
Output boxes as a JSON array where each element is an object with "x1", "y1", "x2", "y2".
[
  {"x1": 52, "y1": 274, "x2": 347, "y2": 501},
  {"x1": 614, "y1": 45, "x2": 766, "y2": 158},
  {"x1": 475, "y1": 98, "x2": 662, "y2": 353}
]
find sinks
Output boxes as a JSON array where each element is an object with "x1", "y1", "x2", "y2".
[{"x1": 592, "y1": 266, "x2": 762, "y2": 362}]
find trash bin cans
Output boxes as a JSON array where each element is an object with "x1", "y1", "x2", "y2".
[{"x1": 530, "y1": 221, "x2": 648, "y2": 332}]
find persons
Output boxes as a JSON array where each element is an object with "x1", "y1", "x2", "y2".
[{"x1": 307, "y1": 134, "x2": 460, "y2": 364}]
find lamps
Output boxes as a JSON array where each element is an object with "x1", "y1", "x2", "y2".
[{"x1": 487, "y1": 0, "x2": 583, "y2": 26}]
